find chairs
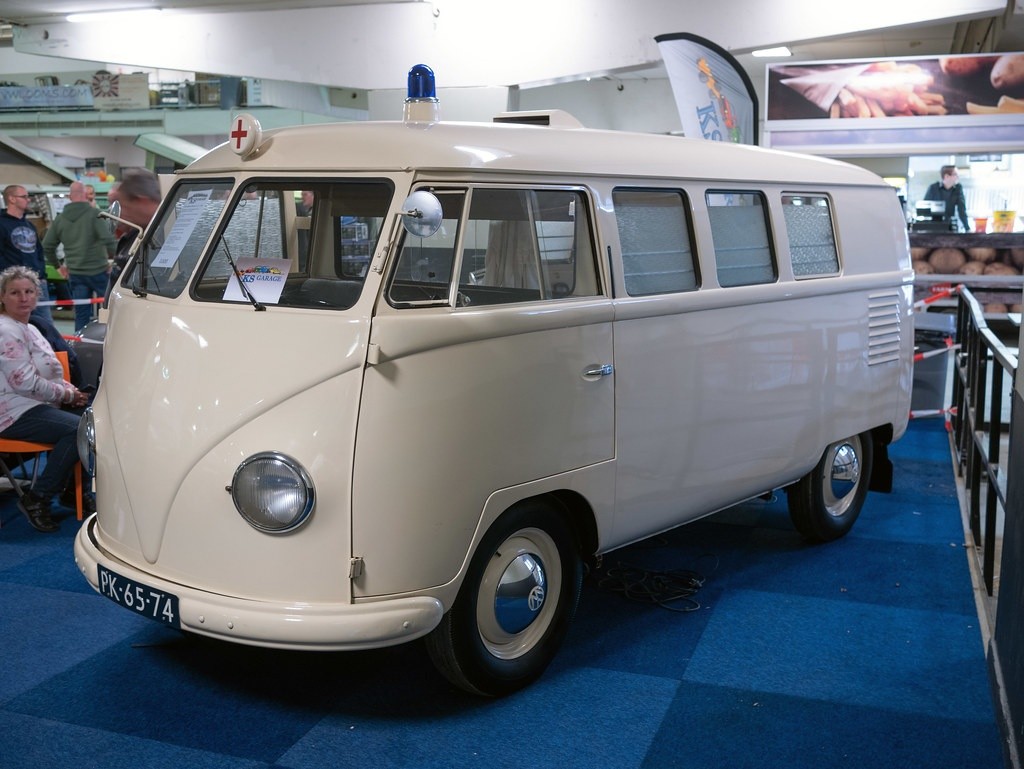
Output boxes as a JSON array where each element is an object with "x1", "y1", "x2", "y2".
[{"x1": 0, "y1": 351, "x2": 84, "y2": 522}]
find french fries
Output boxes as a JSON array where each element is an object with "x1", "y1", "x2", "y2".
[{"x1": 830, "y1": 61, "x2": 1024, "y2": 118}]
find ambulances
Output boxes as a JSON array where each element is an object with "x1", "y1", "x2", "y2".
[{"x1": 72, "y1": 64, "x2": 914, "y2": 698}]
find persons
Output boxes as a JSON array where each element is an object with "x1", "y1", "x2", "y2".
[
  {"x1": 107, "y1": 188, "x2": 121, "y2": 233},
  {"x1": 924, "y1": 162, "x2": 972, "y2": 233},
  {"x1": 0, "y1": 266, "x2": 94, "y2": 532},
  {"x1": 81, "y1": 183, "x2": 100, "y2": 209},
  {"x1": 0, "y1": 185, "x2": 52, "y2": 328},
  {"x1": 102, "y1": 166, "x2": 166, "y2": 309},
  {"x1": 42, "y1": 180, "x2": 116, "y2": 334},
  {"x1": 211, "y1": 187, "x2": 280, "y2": 201},
  {"x1": 303, "y1": 190, "x2": 314, "y2": 259}
]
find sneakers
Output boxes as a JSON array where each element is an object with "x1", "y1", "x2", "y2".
[
  {"x1": 17, "y1": 495, "x2": 60, "y2": 532},
  {"x1": 59, "y1": 487, "x2": 96, "y2": 513}
]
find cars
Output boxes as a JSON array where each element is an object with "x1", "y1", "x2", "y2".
[
  {"x1": 66, "y1": 319, "x2": 105, "y2": 396},
  {"x1": 0, "y1": 185, "x2": 115, "y2": 310}
]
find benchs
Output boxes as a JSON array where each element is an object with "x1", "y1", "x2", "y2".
[{"x1": 299, "y1": 275, "x2": 562, "y2": 310}]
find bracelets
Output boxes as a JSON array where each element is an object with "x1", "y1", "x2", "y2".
[{"x1": 63, "y1": 388, "x2": 74, "y2": 404}]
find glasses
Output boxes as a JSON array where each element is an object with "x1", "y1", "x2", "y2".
[{"x1": 14, "y1": 195, "x2": 29, "y2": 199}]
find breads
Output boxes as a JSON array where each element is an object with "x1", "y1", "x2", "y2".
[{"x1": 910, "y1": 245, "x2": 1023, "y2": 313}]
